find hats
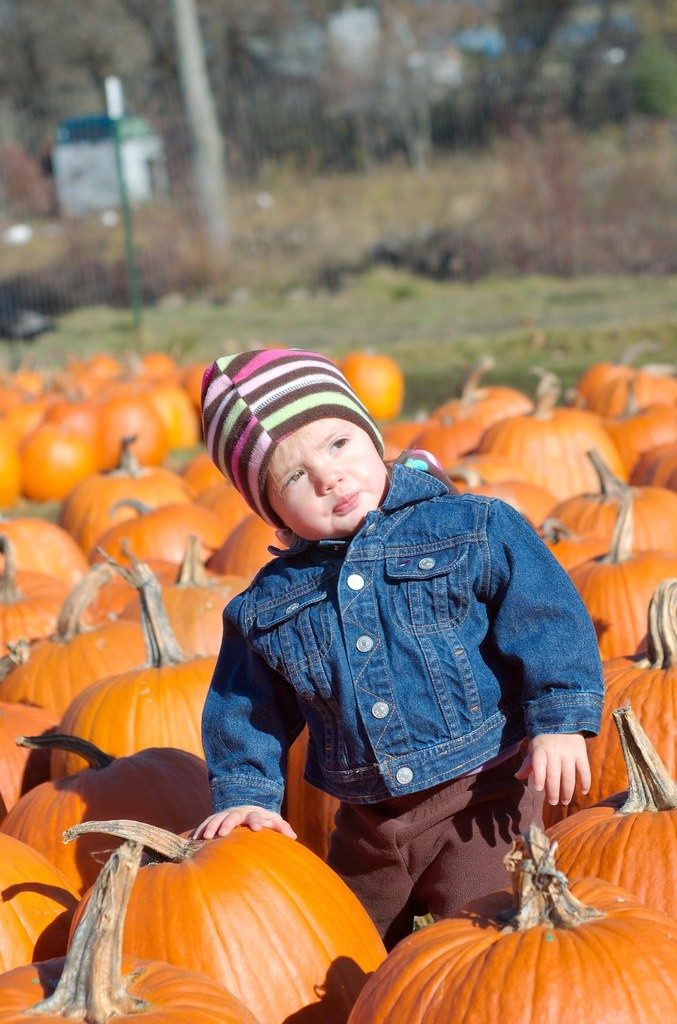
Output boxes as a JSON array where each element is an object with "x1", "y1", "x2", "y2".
[{"x1": 203, "y1": 348, "x2": 384, "y2": 528}]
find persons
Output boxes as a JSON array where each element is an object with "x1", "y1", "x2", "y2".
[{"x1": 191, "y1": 348, "x2": 606, "y2": 952}]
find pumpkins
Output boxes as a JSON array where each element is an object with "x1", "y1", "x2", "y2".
[{"x1": 1, "y1": 342, "x2": 677, "y2": 1024}]
